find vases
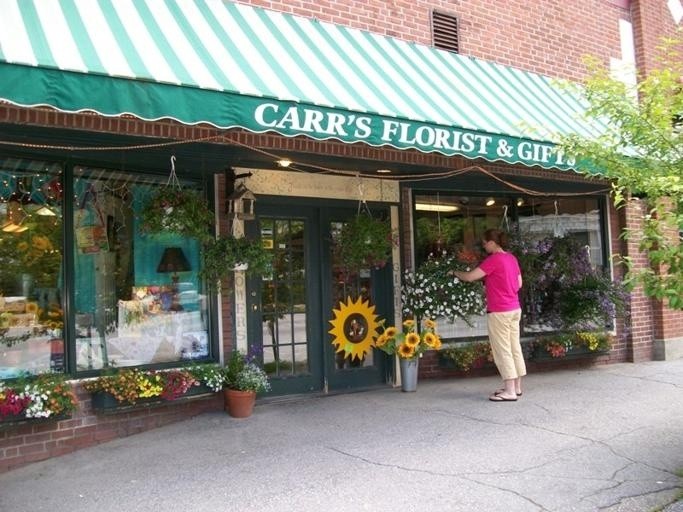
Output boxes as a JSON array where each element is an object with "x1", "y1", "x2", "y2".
[
  {"x1": 162, "y1": 206, "x2": 189, "y2": 232},
  {"x1": 398, "y1": 357, "x2": 420, "y2": 391},
  {"x1": 1, "y1": 410, "x2": 73, "y2": 423}
]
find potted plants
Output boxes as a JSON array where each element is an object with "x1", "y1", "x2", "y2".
[
  {"x1": 332, "y1": 211, "x2": 403, "y2": 273},
  {"x1": 195, "y1": 230, "x2": 272, "y2": 298}
]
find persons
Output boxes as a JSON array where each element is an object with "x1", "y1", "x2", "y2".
[{"x1": 446, "y1": 229, "x2": 527, "y2": 401}]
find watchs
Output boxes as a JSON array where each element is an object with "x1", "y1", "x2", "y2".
[{"x1": 453, "y1": 270, "x2": 456, "y2": 276}]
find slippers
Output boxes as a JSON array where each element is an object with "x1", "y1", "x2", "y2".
[{"x1": 489, "y1": 388, "x2": 522, "y2": 401}]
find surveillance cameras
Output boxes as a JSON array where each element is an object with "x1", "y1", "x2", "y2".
[{"x1": 457, "y1": 196, "x2": 470, "y2": 206}]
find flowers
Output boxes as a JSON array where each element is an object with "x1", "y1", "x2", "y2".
[
  {"x1": 0, "y1": 342, "x2": 273, "y2": 418},
  {"x1": 505, "y1": 221, "x2": 636, "y2": 359},
  {"x1": 0, "y1": 296, "x2": 63, "y2": 346},
  {"x1": 375, "y1": 249, "x2": 493, "y2": 375},
  {"x1": 131, "y1": 186, "x2": 216, "y2": 244}
]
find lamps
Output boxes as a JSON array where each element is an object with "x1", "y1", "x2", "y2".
[{"x1": 157, "y1": 246, "x2": 192, "y2": 313}]
